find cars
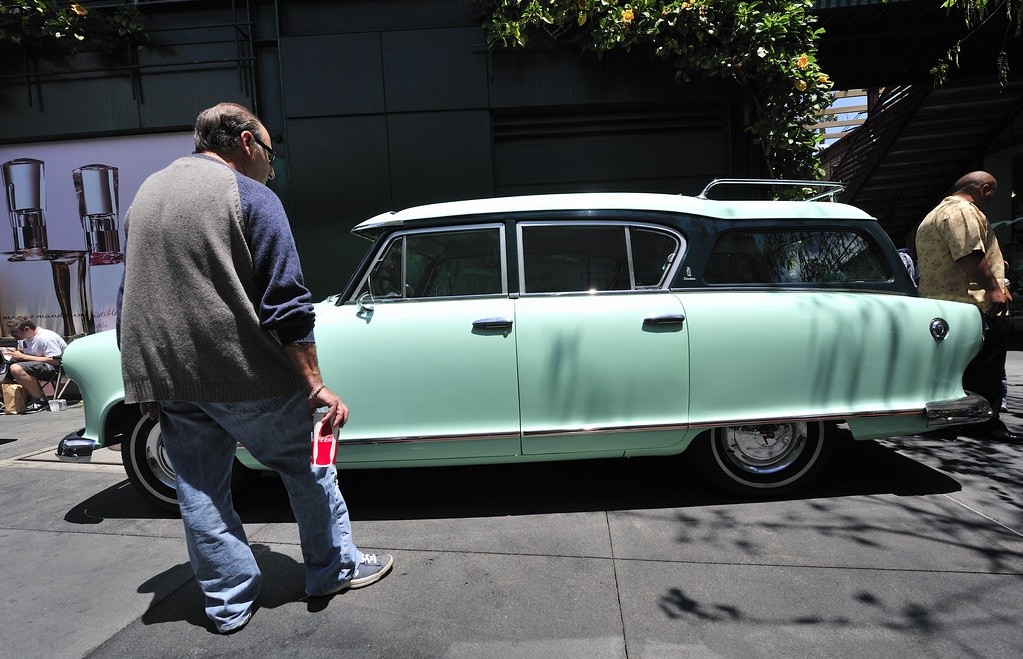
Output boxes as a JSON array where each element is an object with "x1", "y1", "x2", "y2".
[{"x1": 55, "y1": 179, "x2": 994, "y2": 511}]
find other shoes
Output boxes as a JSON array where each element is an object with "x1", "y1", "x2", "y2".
[{"x1": 1000, "y1": 398, "x2": 1008, "y2": 411}]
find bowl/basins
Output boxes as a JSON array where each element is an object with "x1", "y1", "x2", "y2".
[{"x1": 47, "y1": 398, "x2": 67, "y2": 412}]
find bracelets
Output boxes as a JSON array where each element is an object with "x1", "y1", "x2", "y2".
[{"x1": 305, "y1": 381, "x2": 325, "y2": 401}]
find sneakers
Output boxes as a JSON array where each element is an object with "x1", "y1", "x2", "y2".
[
  {"x1": 310, "y1": 553, "x2": 394, "y2": 596},
  {"x1": 21, "y1": 395, "x2": 50, "y2": 414}
]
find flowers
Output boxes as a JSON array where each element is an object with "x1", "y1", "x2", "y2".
[{"x1": 484, "y1": 0, "x2": 834, "y2": 180}]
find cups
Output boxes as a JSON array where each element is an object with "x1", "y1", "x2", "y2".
[{"x1": 309, "y1": 411, "x2": 342, "y2": 469}]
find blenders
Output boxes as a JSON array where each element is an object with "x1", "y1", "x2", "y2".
[
  {"x1": 2, "y1": 156, "x2": 49, "y2": 250},
  {"x1": 70, "y1": 163, "x2": 121, "y2": 251}
]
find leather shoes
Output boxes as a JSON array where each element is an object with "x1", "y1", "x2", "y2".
[{"x1": 976, "y1": 421, "x2": 1023, "y2": 444}]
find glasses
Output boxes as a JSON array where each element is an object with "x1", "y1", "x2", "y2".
[
  {"x1": 238, "y1": 129, "x2": 277, "y2": 166},
  {"x1": 8, "y1": 324, "x2": 32, "y2": 335}
]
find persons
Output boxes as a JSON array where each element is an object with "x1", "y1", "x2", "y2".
[
  {"x1": 894, "y1": 248, "x2": 917, "y2": 289},
  {"x1": 999, "y1": 260, "x2": 1013, "y2": 413},
  {"x1": 915, "y1": 170, "x2": 1011, "y2": 417},
  {"x1": 117, "y1": 103, "x2": 396, "y2": 635},
  {"x1": 5, "y1": 316, "x2": 68, "y2": 414}
]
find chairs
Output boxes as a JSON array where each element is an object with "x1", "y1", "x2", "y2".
[
  {"x1": 473, "y1": 261, "x2": 584, "y2": 293},
  {"x1": 640, "y1": 261, "x2": 671, "y2": 287},
  {"x1": 39, "y1": 333, "x2": 87, "y2": 400}
]
